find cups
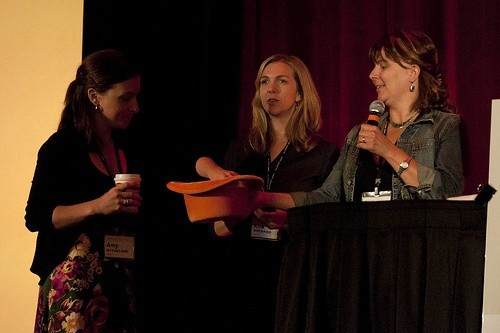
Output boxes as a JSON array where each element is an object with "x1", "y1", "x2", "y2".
[{"x1": 114, "y1": 174, "x2": 141, "y2": 212}]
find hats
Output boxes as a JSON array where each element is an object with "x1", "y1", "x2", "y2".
[{"x1": 168, "y1": 174, "x2": 265, "y2": 227}]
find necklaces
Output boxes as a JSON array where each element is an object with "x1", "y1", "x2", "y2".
[{"x1": 388, "y1": 110, "x2": 419, "y2": 128}]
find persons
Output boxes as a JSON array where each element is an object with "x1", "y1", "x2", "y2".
[
  {"x1": 247, "y1": 28, "x2": 465, "y2": 215},
  {"x1": 25, "y1": 50, "x2": 239, "y2": 333},
  {"x1": 194, "y1": 54, "x2": 340, "y2": 333}
]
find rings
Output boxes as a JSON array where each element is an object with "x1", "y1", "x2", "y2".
[
  {"x1": 363, "y1": 137, "x2": 365, "y2": 142},
  {"x1": 124, "y1": 199, "x2": 128, "y2": 205}
]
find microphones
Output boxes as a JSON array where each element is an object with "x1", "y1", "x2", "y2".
[{"x1": 355, "y1": 100, "x2": 385, "y2": 167}]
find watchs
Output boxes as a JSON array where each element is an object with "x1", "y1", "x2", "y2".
[{"x1": 397, "y1": 156, "x2": 413, "y2": 176}]
